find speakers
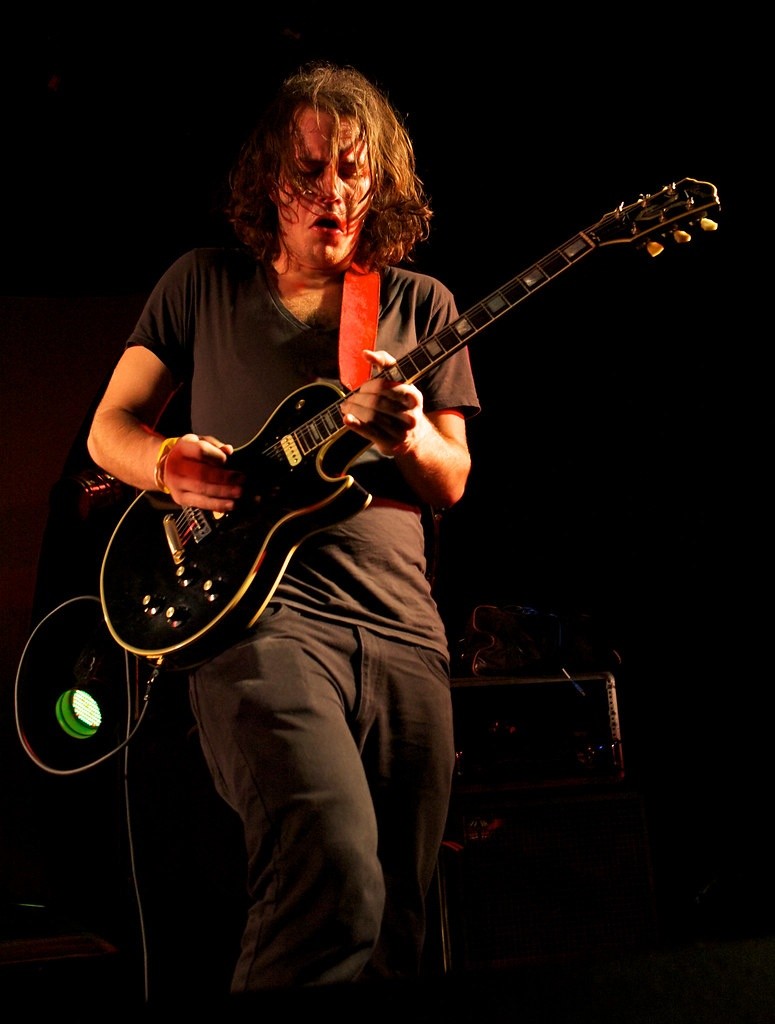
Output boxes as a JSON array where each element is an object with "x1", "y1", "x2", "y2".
[{"x1": 435, "y1": 789, "x2": 661, "y2": 974}]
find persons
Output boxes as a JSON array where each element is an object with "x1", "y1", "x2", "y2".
[{"x1": 87, "y1": 61, "x2": 481, "y2": 1023}]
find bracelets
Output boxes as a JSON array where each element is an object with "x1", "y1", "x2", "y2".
[{"x1": 153, "y1": 436, "x2": 179, "y2": 494}]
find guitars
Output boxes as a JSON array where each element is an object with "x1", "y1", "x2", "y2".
[{"x1": 99, "y1": 175, "x2": 723, "y2": 669}]
